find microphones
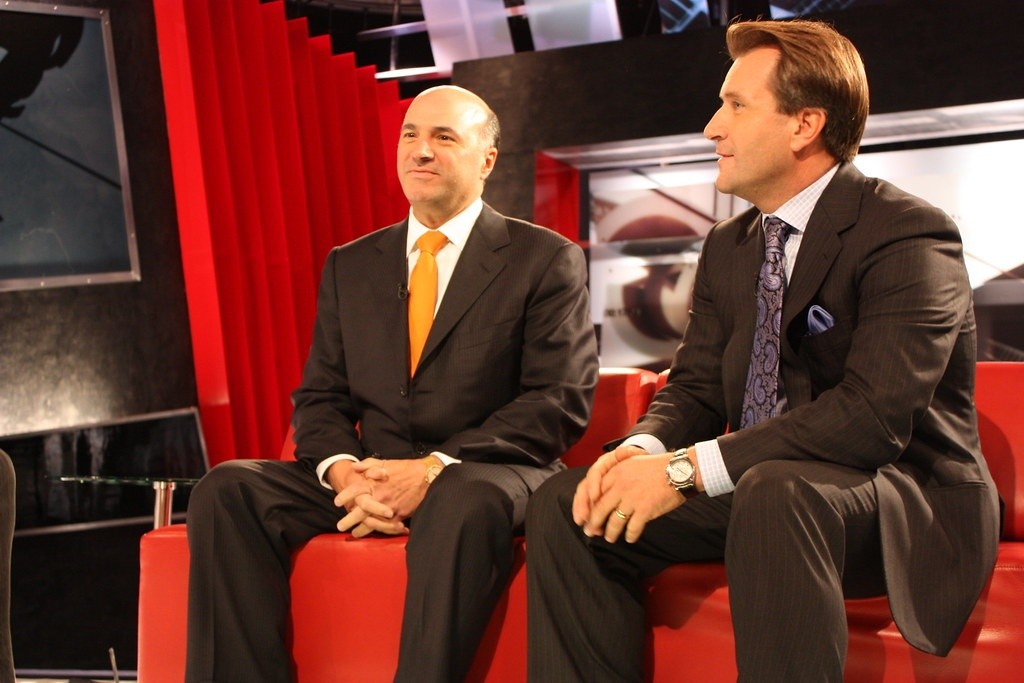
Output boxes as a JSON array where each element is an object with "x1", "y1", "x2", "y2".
[{"x1": 398, "y1": 281, "x2": 405, "y2": 290}]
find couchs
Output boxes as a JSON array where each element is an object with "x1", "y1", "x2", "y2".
[{"x1": 139, "y1": 360, "x2": 1024, "y2": 683}]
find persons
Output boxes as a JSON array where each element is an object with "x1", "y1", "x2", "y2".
[
  {"x1": 183, "y1": 85, "x2": 599, "y2": 683},
  {"x1": 527, "y1": 20, "x2": 1000, "y2": 683}
]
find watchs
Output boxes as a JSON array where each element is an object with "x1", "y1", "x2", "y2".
[
  {"x1": 666, "y1": 448, "x2": 699, "y2": 498},
  {"x1": 425, "y1": 456, "x2": 442, "y2": 483}
]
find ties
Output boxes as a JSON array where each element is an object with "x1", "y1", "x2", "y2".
[
  {"x1": 740, "y1": 217, "x2": 793, "y2": 432},
  {"x1": 407, "y1": 230, "x2": 449, "y2": 382}
]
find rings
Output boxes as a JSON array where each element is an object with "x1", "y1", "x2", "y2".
[{"x1": 615, "y1": 508, "x2": 630, "y2": 519}]
